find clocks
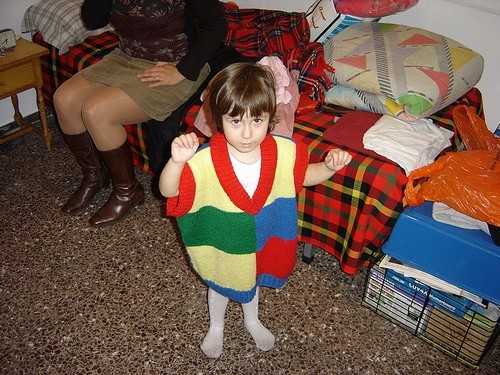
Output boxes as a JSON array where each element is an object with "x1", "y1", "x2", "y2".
[{"x1": 0, "y1": 28, "x2": 16, "y2": 49}]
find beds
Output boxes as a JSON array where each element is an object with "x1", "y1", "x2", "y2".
[{"x1": 30, "y1": 26, "x2": 485, "y2": 276}]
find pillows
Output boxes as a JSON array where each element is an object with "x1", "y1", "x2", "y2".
[{"x1": 21, "y1": 0, "x2": 115, "y2": 55}]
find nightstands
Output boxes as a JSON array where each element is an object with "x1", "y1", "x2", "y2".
[{"x1": 0, "y1": 38, "x2": 53, "y2": 151}]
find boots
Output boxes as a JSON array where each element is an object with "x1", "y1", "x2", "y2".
[
  {"x1": 60, "y1": 129, "x2": 111, "y2": 215},
  {"x1": 89, "y1": 136, "x2": 146, "y2": 227}
]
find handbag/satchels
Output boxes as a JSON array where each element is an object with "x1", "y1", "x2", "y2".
[
  {"x1": 404, "y1": 146, "x2": 499, "y2": 227},
  {"x1": 451, "y1": 104, "x2": 499, "y2": 150}
]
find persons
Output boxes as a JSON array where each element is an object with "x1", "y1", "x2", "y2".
[
  {"x1": 53, "y1": 0, "x2": 228, "y2": 227},
  {"x1": 158, "y1": 62, "x2": 352, "y2": 359}
]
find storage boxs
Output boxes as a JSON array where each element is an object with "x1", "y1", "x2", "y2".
[{"x1": 380, "y1": 201, "x2": 500, "y2": 305}]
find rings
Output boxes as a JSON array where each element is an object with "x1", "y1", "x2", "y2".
[{"x1": 157, "y1": 76, "x2": 160, "y2": 81}]
